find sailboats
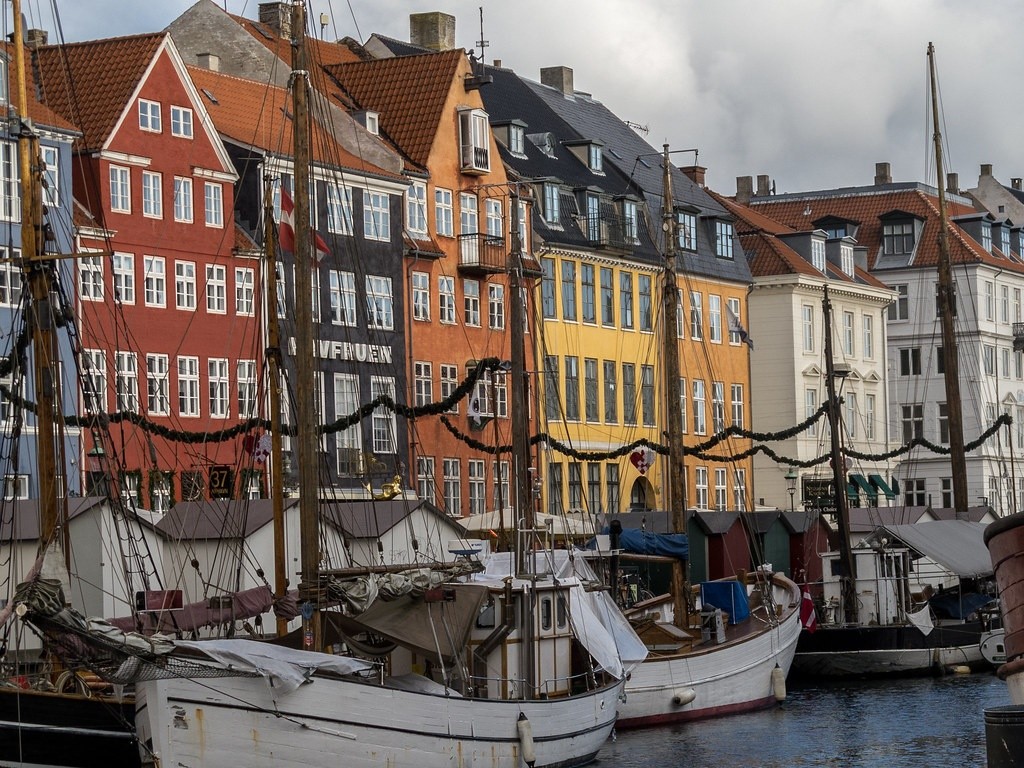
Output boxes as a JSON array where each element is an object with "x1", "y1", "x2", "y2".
[{"x1": 3, "y1": 0, "x2": 1023, "y2": 768}]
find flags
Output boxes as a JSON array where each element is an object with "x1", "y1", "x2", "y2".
[
  {"x1": 278, "y1": 185, "x2": 331, "y2": 271},
  {"x1": 467, "y1": 383, "x2": 481, "y2": 429},
  {"x1": 799, "y1": 581, "x2": 818, "y2": 636}
]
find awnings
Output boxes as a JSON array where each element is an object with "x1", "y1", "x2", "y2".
[{"x1": 830, "y1": 475, "x2": 895, "y2": 501}]
